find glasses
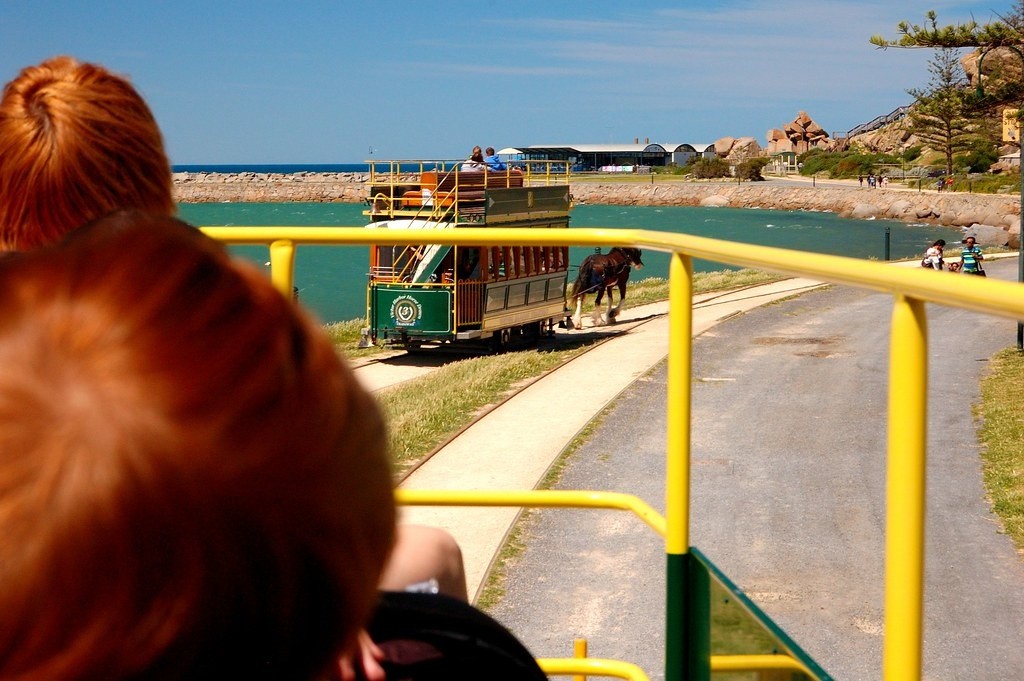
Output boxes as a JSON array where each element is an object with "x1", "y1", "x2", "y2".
[{"x1": 965, "y1": 241, "x2": 973, "y2": 244}]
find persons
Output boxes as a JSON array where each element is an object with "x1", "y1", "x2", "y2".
[
  {"x1": 1, "y1": 211, "x2": 398, "y2": 680},
  {"x1": 461, "y1": 154, "x2": 496, "y2": 173},
  {"x1": 949, "y1": 263, "x2": 959, "y2": 272},
  {"x1": 856, "y1": 174, "x2": 955, "y2": 195},
  {"x1": 958, "y1": 237, "x2": 983, "y2": 274},
  {"x1": 484, "y1": 147, "x2": 508, "y2": 172},
  {"x1": 922, "y1": 239, "x2": 945, "y2": 271},
  {"x1": 488, "y1": 251, "x2": 506, "y2": 277},
  {"x1": 469, "y1": 146, "x2": 481, "y2": 160},
  {"x1": 1, "y1": 59, "x2": 173, "y2": 253},
  {"x1": 927, "y1": 241, "x2": 940, "y2": 270}
]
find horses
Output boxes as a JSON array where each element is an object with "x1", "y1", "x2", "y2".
[{"x1": 567, "y1": 246, "x2": 644, "y2": 329}]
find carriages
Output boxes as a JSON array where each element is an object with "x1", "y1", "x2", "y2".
[{"x1": 362, "y1": 156, "x2": 646, "y2": 356}]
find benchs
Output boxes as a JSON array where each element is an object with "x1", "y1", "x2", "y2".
[{"x1": 402, "y1": 170, "x2": 523, "y2": 207}]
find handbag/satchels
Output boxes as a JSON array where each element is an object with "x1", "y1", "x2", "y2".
[
  {"x1": 921, "y1": 257, "x2": 933, "y2": 269},
  {"x1": 972, "y1": 269, "x2": 986, "y2": 277}
]
